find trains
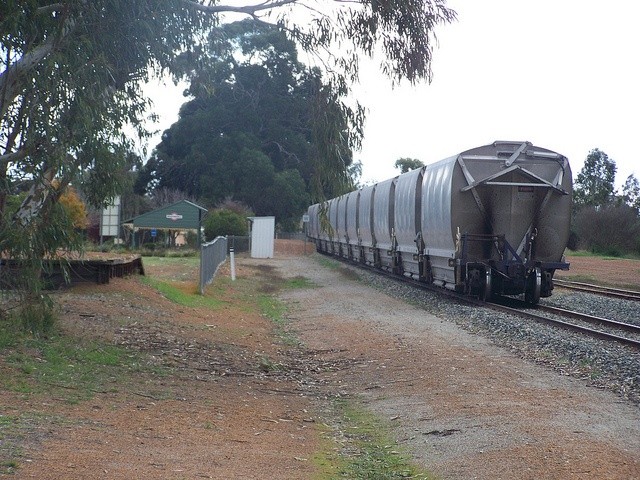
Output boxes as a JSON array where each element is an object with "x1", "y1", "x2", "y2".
[{"x1": 308, "y1": 139, "x2": 574, "y2": 307}]
